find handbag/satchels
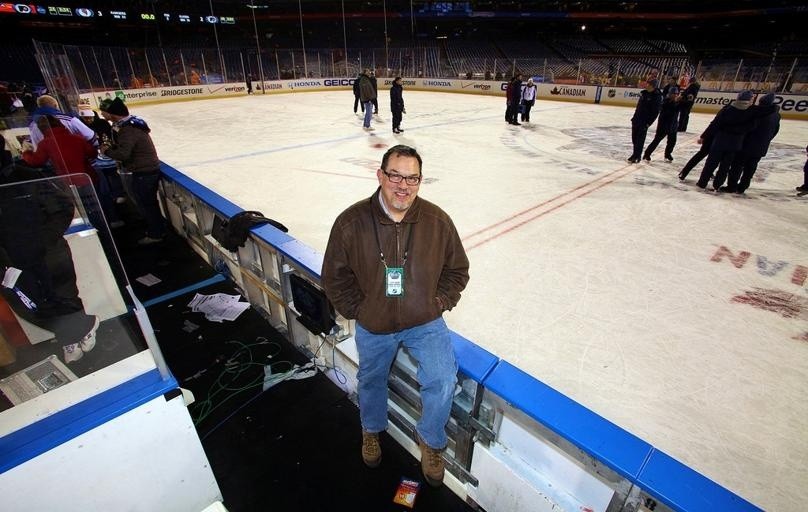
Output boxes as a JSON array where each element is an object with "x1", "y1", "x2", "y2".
[{"x1": 517, "y1": 102, "x2": 526, "y2": 114}]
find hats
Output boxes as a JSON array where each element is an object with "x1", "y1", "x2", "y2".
[
  {"x1": 736, "y1": 90, "x2": 753, "y2": 100},
  {"x1": 107, "y1": 97, "x2": 130, "y2": 116},
  {"x1": 668, "y1": 87, "x2": 679, "y2": 94},
  {"x1": 99, "y1": 99, "x2": 113, "y2": 113},
  {"x1": 758, "y1": 93, "x2": 774, "y2": 105},
  {"x1": 78, "y1": 108, "x2": 94, "y2": 116}
]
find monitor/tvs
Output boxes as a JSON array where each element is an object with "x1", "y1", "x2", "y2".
[{"x1": 289, "y1": 273, "x2": 335, "y2": 335}]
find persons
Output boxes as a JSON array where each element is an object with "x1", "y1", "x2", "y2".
[
  {"x1": 629, "y1": 66, "x2": 807, "y2": 199},
  {"x1": 1, "y1": 135, "x2": 101, "y2": 363},
  {"x1": 1, "y1": 78, "x2": 168, "y2": 267},
  {"x1": 504, "y1": 74, "x2": 538, "y2": 126},
  {"x1": 318, "y1": 145, "x2": 472, "y2": 490},
  {"x1": 349, "y1": 68, "x2": 379, "y2": 132},
  {"x1": 105, "y1": 51, "x2": 258, "y2": 97},
  {"x1": 389, "y1": 77, "x2": 407, "y2": 133}
]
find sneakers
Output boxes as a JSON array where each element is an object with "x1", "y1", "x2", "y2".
[
  {"x1": 62, "y1": 343, "x2": 84, "y2": 364},
  {"x1": 137, "y1": 236, "x2": 162, "y2": 244},
  {"x1": 413, "y1": 428, "x2": 447, "y2": 488},
  {"x1": 79, "y1": 315, "x2": 99, "y2": 353},
  {"x1": 664, "y1": 153, "x2": 673, "y2": 160},
  {"x1": 361, "y1": 429, "x2": 382, "y2": 469},
  {"x1": 110, "y1": 220, "x2": 124, "y2": 228}
]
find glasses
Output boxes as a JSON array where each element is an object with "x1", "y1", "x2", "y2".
[{"x1": 384, "y1": 172, "x2": 420, "y2": 186}]
